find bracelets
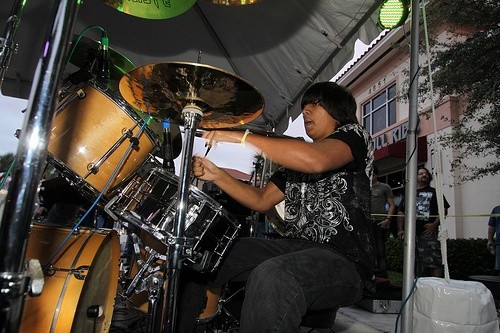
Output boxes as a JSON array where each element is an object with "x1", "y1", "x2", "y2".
[
  {"x1": 241, "y1": 129, "x2": 253, "y2": 144},
  {"x1": 386, "y1": 218, "x2": 391, "y2": 221}
]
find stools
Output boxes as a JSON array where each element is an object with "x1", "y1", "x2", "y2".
[{"x1": 222, "y1": 285, "x2": 337, "y2": 330}]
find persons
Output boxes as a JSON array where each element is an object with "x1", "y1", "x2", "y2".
[
  {"x1": 371, "y1": 165, "x2": 395, "y2": 278},
  {"x1": 397, "y1": 167, "x2": 451, "y2": 278},
  {"x1": 192, "y1": 81, "x2": 376, "y2": 333},
  {"x1": 487, "y1": 206, "x2": 500, "y2": 270}
]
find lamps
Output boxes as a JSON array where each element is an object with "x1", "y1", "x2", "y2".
[{"x1": 377, "y1": 0, "x2": 409, "y2": 29}]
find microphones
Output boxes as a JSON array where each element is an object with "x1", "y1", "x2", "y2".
[
  {"x1": 162, "y1": 118, "x2": 176, "y2": 174},
  {"x1": 95, "y1": 32, "x2": 109, "y2": 88}
]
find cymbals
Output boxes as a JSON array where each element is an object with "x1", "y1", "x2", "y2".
[
  {"x1": 118, "y1": 61, "x2": 264, "y2": 129},
  {"x1": 68, "y1": 32, "x2": 136, "y2": 80}
]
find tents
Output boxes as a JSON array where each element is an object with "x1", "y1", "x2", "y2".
[{"x1": 0, "y1": 0, "x2": 419, "y2": 333}]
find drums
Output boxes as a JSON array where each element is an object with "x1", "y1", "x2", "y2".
[
  {"x1": 15, "y1": 222, "x2": 121, "y2": 333},
  {"x1": 124, "y1": 235, "x2": 224, "y2": 323},
  {"x1": 14, "y1": 79, "x2": 160, "y2": 208},
  {"x1": 105, "y1": 165, "x2": 242, "y2": 276}
]
